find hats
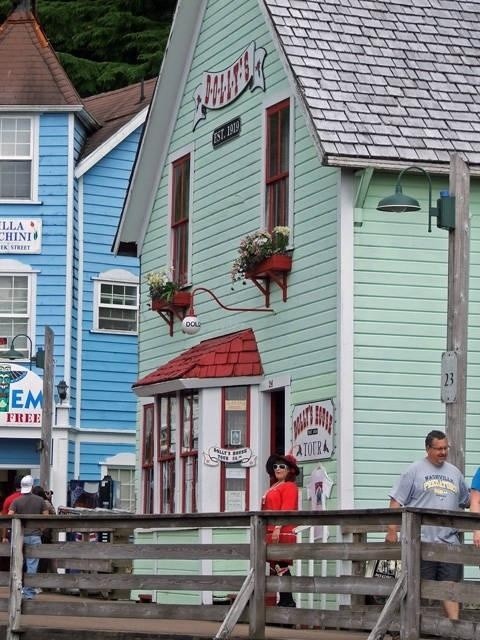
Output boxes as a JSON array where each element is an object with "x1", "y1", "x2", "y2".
[
  {"x1": 265, "y1": 454, "x2": 300, "y2": 481},
  {"x1": 20, "y1": 475, "x2": 35, "y2": 494}
]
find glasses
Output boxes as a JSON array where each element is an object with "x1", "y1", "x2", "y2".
[
  {"x1": 431, "y1": 445, "x2": 452, "y2": 451},
  {"x1": 271, "y1": 463, "x2": 288, "y2": 470}
]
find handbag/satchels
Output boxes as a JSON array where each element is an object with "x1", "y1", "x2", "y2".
[{"x1": 362, "y1": 546, "x2": 407, "y2": 606}]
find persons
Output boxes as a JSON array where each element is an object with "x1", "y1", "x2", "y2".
[
  {"x1": 260, "y1": 454, "x2": 299, "y2": 608},
  {"x1": 386, "y1": 430, "x2": 471, "y2": 620},
  {"x1": 0, "y1": 475, "x2": 57, "y2": 600},
  {"x1": 469, "y1": 467, "x2": 480, "y2": 548}
]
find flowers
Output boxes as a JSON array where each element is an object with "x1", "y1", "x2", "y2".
[
  {"x1": 145, "y1": 266, "x2": 181, "y2": 306},
  {"x1": 230, "y1": 224, "x2": 291, "y2": 293}
]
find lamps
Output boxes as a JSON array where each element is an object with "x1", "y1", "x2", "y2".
[
  {"x1": 54, "y1": 379, "x2": 68, "y2": 405},
  {"x1": 180, "y1": 286, "x2": 273, "y2": 337}
]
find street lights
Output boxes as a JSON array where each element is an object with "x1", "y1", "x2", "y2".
[
  {"x1": 0, "y1": 324, "x2": 53, "y2": 492},
  {"x1": 374, "y1": 152, "x2": 469, "y2": 481}
]
